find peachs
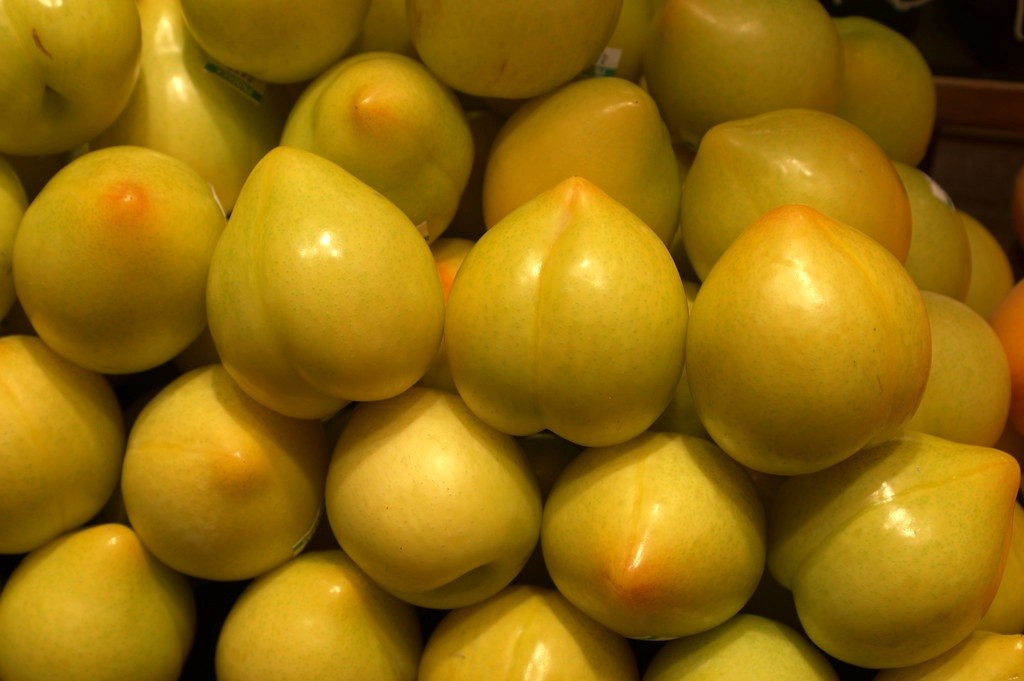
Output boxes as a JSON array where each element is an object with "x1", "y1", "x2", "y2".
[{"x1": 0, "y1": 0, "x2": 1024, "y2": 681}]
